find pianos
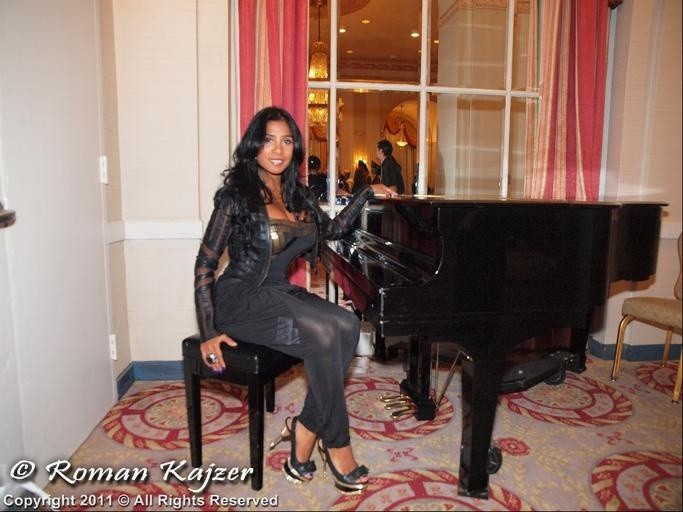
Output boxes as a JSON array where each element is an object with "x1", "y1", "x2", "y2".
[{"x1": 321, "y1": 196, "x2": 670, "y2": 500}]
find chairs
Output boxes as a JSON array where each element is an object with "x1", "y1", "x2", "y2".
[{"x1": 612, "y1": 232, "x2": 683, "y2": 405}]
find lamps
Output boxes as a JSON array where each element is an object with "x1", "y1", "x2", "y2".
[{"x1": 308, "y1": 1, "x2": 328, "y2": 126}]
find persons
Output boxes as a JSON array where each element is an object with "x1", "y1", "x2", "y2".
[
  {"x1": 308, "y1": 140, "x2": 404, "y2": 202},
  {"x1": 194, "y1": 108, "x2": 397, "y2": 496}
]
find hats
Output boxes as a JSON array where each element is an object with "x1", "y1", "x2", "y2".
[{"x1": 308, "y1": 155, "x2": 321, "y2": 170}]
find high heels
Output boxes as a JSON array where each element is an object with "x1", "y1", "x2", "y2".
[
  {"x1": 317, "y1": 438, "x2": 369, "y2": 495},
  {"x1": 269, "y1": 417, "x2": 316, "y2": 484}
]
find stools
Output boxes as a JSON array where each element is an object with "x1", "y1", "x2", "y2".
[{"x1": 182, "y1": 330, "x2": 304, "y2": 490}]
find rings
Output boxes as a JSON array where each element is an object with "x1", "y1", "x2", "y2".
[{"x1": 207, "y1": 353, "x2": 218, "y2": 364}]
